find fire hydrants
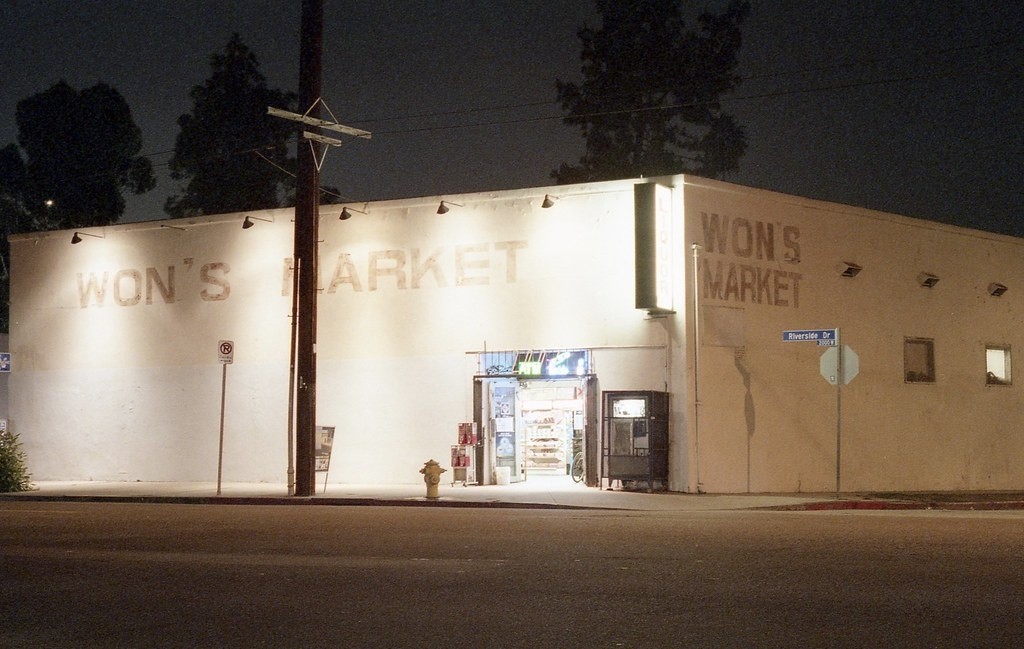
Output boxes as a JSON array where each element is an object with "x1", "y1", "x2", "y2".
[{"x1": 419, "y1": 458, "x2": 448, "y2": 499}]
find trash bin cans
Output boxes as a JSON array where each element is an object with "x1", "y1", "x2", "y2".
[{"x1": 496, "y1": 466, "x2": 511, "y2": 485}]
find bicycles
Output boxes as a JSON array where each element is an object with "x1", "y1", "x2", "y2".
[{"x1": 571, "y1": 437, "x2": 585, "y2": 482}]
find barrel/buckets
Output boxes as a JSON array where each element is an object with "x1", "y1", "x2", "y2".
[{"x1": 496, "y1": 467, "x2": 511, "y2": 485}]
